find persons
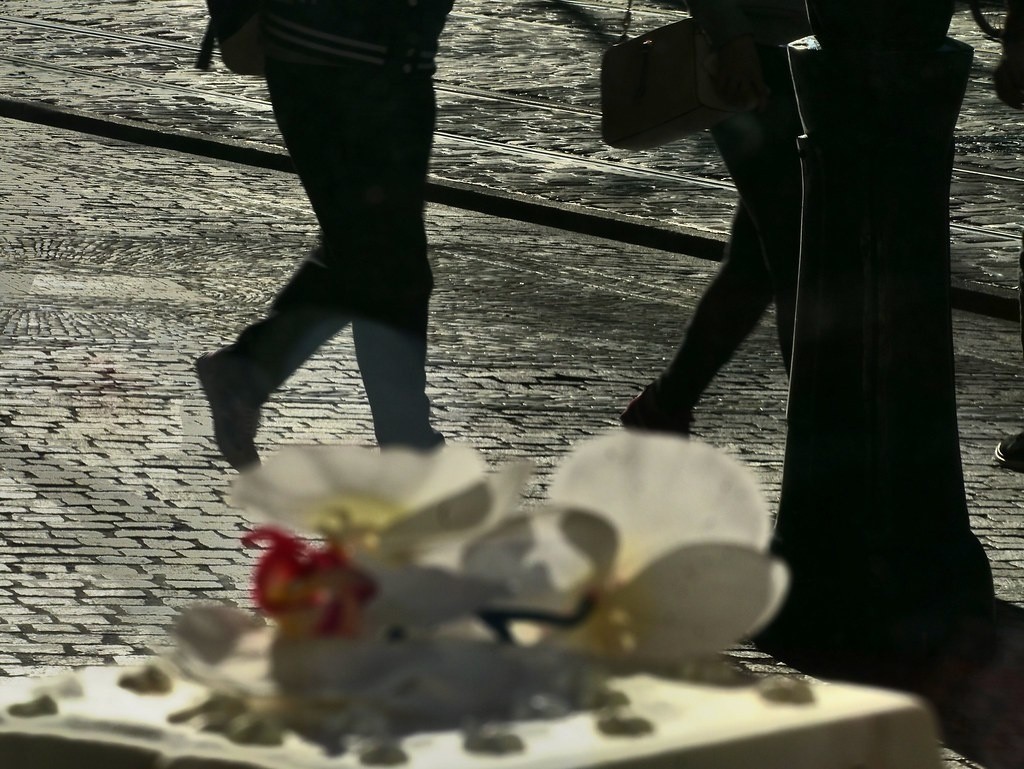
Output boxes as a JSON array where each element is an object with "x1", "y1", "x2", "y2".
[
  {"x1": 620, "y1": 0, "x2": 829, "y2": 442},
  {"x1": 191, "y1": 0, "x2": 457, "y2": 471}
]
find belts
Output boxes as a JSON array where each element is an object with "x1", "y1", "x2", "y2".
[{"x1": 265, "y1": 16, "x2": 438, "y2": 70}]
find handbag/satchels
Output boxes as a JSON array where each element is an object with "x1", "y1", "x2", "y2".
[
  {"x1": 195, "y1": 0, "x2": 262, "y2": 75},
  {"x1": 600, "y1": 0, "x2": 708, "y2": 150}
]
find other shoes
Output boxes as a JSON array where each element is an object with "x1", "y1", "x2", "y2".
[
  {"x1": 619, "y1": 381, "x2": 694, "y2": 440},
  {"x1": 195, "y1": 345, "x2": 266, "y2": 472},
  {"x1": 994, "y1": 432, "x2": 1024, "y2": 471}
]
